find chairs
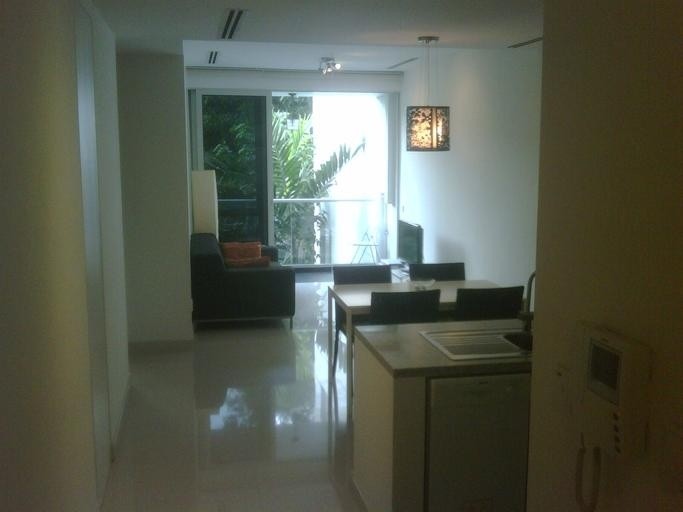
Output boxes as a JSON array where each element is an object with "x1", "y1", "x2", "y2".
[
  {"x1": 369, "y1": 289, "x2": 441, "y2": 325},
  {"x1": 453, "y1": 286, "x2": 525, "y2": 321},
  {"x1": 333, "y1": 264, "x2": 393, "y2": 286},
  {"x1": 409, "y1": 262, "x2": 466, "y2": 282}
]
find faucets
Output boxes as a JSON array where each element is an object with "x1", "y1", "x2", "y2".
[{"x1": 517, "y1": 271, "x2": 535, "y2": 331}]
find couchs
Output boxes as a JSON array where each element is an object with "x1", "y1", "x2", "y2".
[{"x1": 190, "y1": 232, "x2": 295, "y2": 329}]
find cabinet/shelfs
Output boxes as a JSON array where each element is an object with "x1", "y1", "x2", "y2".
[{"x1": 351, "y1": 318, "x2": 532, "y2": 512}]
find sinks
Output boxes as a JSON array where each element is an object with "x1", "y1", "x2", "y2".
[{"x1": 503, "y1": 328, "x2": 533, "y2": 358}]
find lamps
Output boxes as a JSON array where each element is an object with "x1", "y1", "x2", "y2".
[
  {"x1": 405, "y1": 36, "x2": 450, "y2": 152},
  {"x1": 319, "y1": 57, "x2": 342, "y2": 78}
]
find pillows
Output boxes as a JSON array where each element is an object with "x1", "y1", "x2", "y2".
[
  {"x1": 219, "y1": 242, "x2": 262, "y2": 258},
  {"x1": 226, "y1": 255, "x2": 271, "y2": 269}
]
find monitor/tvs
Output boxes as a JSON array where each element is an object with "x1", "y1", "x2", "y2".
[{"x1": 394, "y1": 219, "x2": 424, "y2": 274}]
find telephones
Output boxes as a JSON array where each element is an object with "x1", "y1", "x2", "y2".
[{"x1": 571, "y1": 320, "x2": 652, "y2": 511}]
